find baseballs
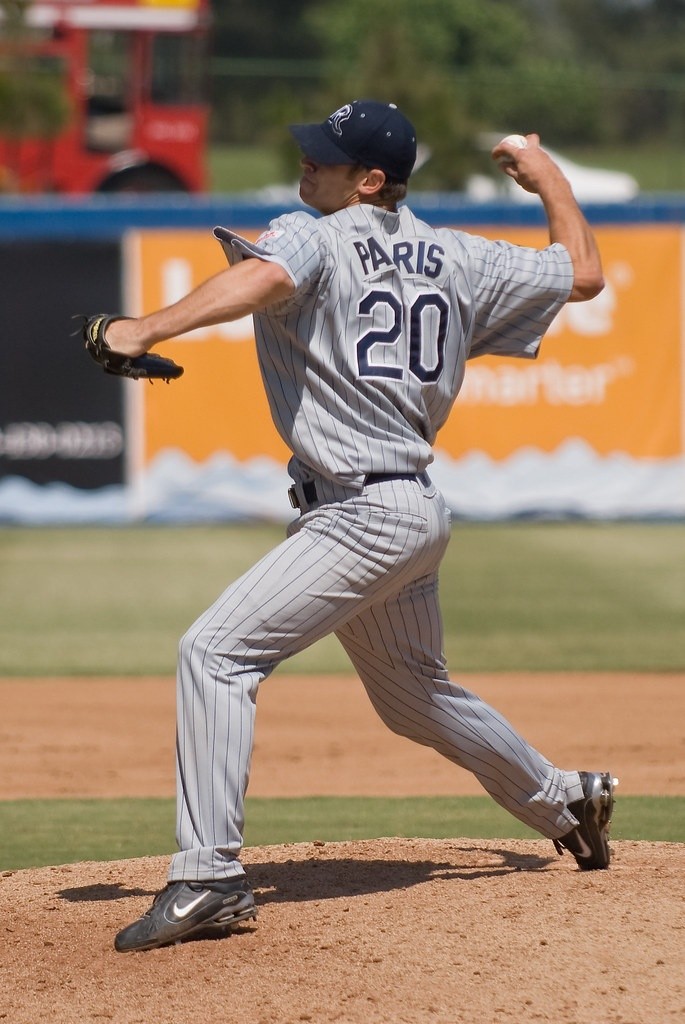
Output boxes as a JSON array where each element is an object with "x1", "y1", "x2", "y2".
[{"x1": 496, "y1": 135, "x2": 528, "y2": 165}]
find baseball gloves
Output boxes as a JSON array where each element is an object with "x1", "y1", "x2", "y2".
[{"x1": 82, "y1": 313, "x2": 184, "y2": 379}]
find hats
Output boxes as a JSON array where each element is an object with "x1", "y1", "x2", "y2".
[{"x1": 281, "y1": 100, "x2": 417, "y2": 181}]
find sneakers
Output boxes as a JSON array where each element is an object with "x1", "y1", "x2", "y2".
[
  {"x1": 113, "y1": 881, "x2": 265, "y2": 952},
  {"x1": 548, "y1": 770, "x2": 621, "y2": 872}
]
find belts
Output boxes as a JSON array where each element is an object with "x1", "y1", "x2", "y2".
[{"x1": 289, "y1": 473, "x2": 416, "y2": 510}]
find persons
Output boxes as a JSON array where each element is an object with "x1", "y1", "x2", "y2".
[
  {"x1": 69, "y1": 100, "x2": 620, "y2": 952},
  {"x1": 80, "y1": 95, "x2": 188, "y2": 194}
]
